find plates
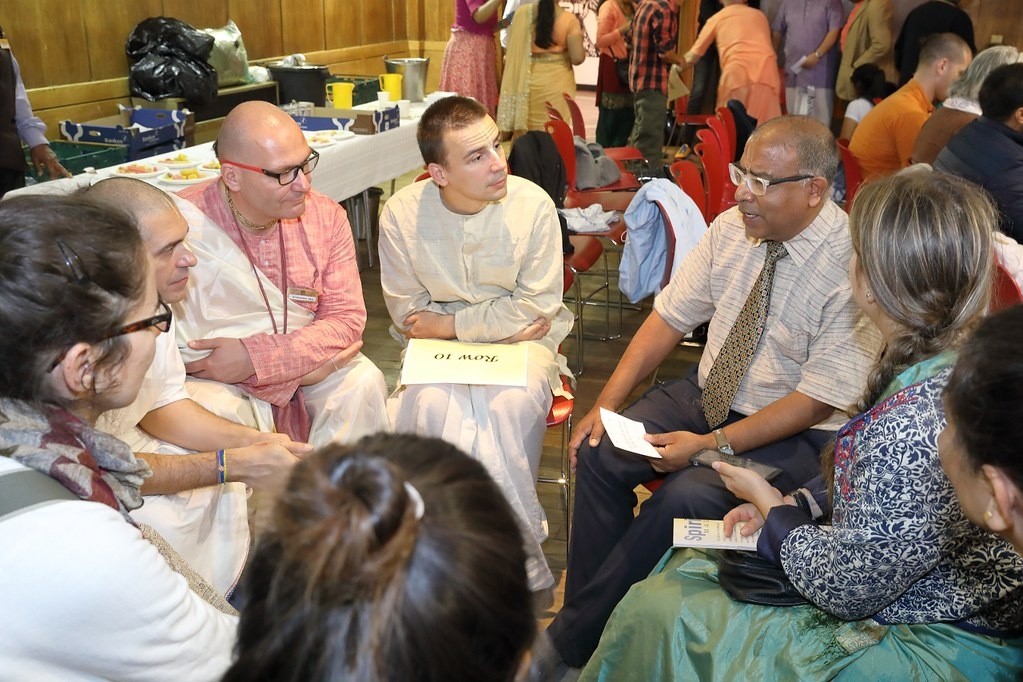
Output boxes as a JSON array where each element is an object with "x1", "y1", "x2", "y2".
[
  {"x1": 109, "y1": 160, "x2": 221, "y2": 185},
  {"x1": 305, "y1": 130, "x2": 355, "y2": 149}
]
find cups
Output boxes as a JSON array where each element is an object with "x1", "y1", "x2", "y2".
[
  {"x1": 379, "y1": 74, "x2": 403, "y2": 101},
  {"x1": 326, "y1": 81, "x2": 355, "y2": 110},
  {"x1": 376, "y1": 92, "x2": 389, "y2": 107}
]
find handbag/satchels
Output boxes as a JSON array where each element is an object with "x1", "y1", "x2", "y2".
[
  {"x1": 718, "y1": 547, "x2": 807, "y2": 609},
  {"x1": 615, "y1": 56, "x2": 630, "y2": 90}
]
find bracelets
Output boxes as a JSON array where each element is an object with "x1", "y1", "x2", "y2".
[
  {"x1": 218, "y1": 449, "x2": 226, "y2": 485},
  {"x1": 786, "y1": 489, "x2": 808, "y2": 508}
]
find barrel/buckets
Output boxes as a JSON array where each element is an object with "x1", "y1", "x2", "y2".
[
  {"x1": 267, "y1": 63, "x2": 328, "y2": 109},
  {"x1": 383, "y1": 55, "x2": 430, "y2": 103}
]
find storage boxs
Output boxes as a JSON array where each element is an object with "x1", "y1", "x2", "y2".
[
  {"x1": 22, "y1": 104, "x2": 186, "y2": 184},
  {"x1": 289, "y1": 107, "x2": 400, "y2": 135}
]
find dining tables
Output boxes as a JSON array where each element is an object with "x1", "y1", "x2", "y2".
[{"x1": 5, "y1": 100, "x2": 478, "y2": 271}]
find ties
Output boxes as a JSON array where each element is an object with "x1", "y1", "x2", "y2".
[{"x1": 703, "y1": 240, "x2": 789, "y2": 430}]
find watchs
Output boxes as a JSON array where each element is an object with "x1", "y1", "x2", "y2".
[
  {"x1": 815, "y1": 49, "x2": 823, "y2": 59},
  {"x1": 713, "y1": 428, "x2": 735, "y2": 455}
]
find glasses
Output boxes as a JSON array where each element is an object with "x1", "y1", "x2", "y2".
[
  {"x1": 47, "y1": 301, "x2": 172, "y2": 373},
  {"x1": 728, "y1": 163, "x2": 814, "y2": 197},
  {"x1": 220, "y1": 147, "x2": 319, "y2": 186}
]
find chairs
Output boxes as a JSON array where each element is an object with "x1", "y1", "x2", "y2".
[{"x1": 414, "y1": 92, "x2": 1023, "y2": 569}]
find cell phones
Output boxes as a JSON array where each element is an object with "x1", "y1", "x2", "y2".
[{"x1": 688, "y1": 448, "x2": 784, "y2": 482}]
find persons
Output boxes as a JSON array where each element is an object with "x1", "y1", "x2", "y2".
[
  {"x1": 0, "y1": 95, "x2": 576, "y2": 682},
  {"x1": 520, "y1": 115, "x2": 1023, "y2": 682},
  {"x1": 439, "y1": 0, "x2": 1023, "y2": 245},
  {"x1": 0, "y1": 25, "x2": 73, "y2": 198}
]
